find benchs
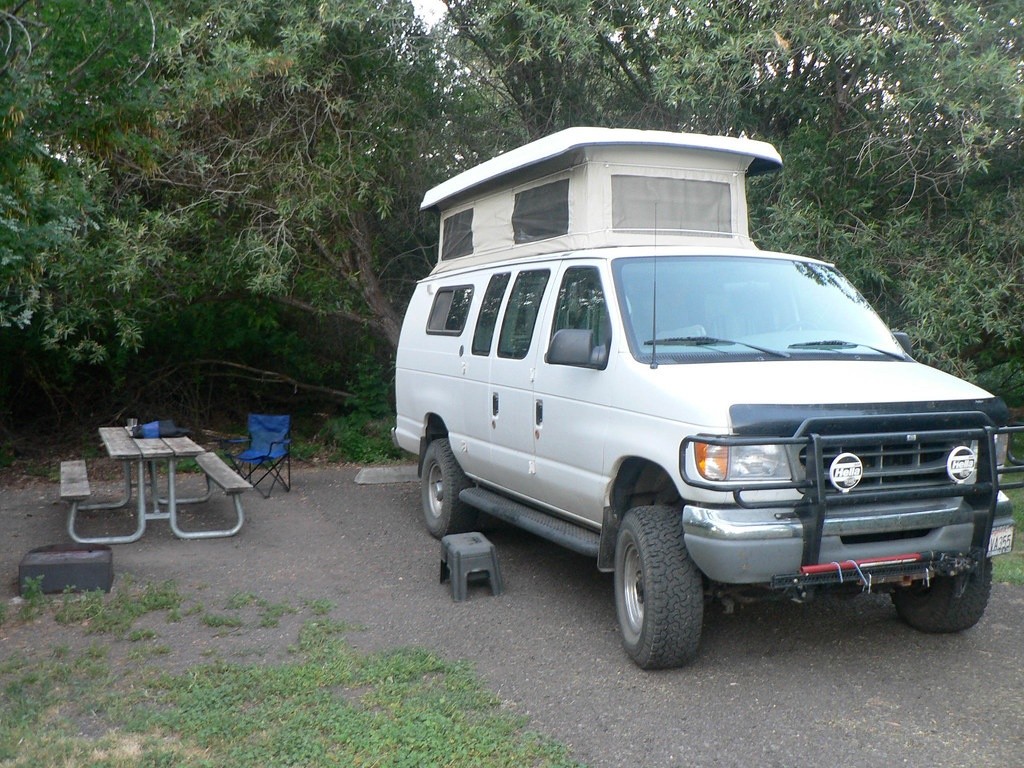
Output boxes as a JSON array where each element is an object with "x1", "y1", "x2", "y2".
[
  {"x1": 196, "y1": 452, "x2": 254, "y2": 495},
  {"x1": 59, "y1": 459, "x2": 91, "y2": 503}
]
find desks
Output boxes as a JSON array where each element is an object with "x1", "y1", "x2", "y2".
[{"x1": 98, "y1": 426, "x2": 206, "y2": 538}]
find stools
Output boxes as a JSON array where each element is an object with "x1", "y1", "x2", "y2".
[{"x1": 438, "y1": 531, "x2": 504, "y2": 604}]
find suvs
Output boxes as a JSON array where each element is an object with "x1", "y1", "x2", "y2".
[{"x1": 391, "y1": 126, "x2": 1014, "y2": 672}]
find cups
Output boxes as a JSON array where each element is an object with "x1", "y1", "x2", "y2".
[{"x1": 128, "y1": 419, "x2": 137, "y2": 431}]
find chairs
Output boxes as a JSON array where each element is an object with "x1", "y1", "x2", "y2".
[{"x1": 218, "y1": 414, "x2": 293, "y2": 497}]
